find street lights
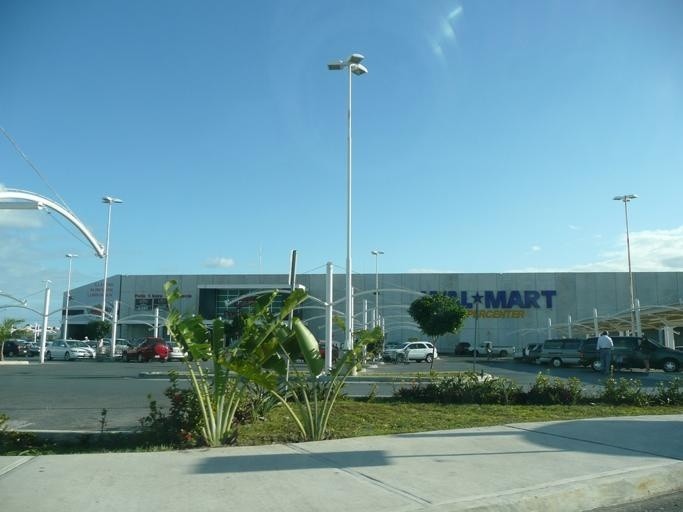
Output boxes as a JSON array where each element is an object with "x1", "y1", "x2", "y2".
[
  {"x1": 327, "y1": 54, "x2": 368, "y2": 377},
  {"x1": 371, "y1": 251, "x2": 384, "y2": 328},
  {"x1": 100, "y1": 197, "x2": 123, "y2": 322},
  {"x1": 614, "y1": 195, "x2": 639, "y2": 310},
  {"x1": 63, "y1": 254, "x2": 77, "y2": 340}
]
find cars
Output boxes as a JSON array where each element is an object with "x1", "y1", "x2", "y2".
[{"x1": 3, "y1": 338, "x2": 213, "y2": 362}]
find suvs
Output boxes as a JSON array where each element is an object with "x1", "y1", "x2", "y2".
[{"x1": 382, "y1": 341, "x2": 438, "y2": 363}]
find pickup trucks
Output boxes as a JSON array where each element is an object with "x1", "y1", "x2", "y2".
[{"x1": 468, "y1": 341, "x2": 515, "y2": 357}]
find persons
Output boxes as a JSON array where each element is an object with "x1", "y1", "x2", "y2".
[
  {"x1": 84, "y1": 336, "x2": 88, "y2": 340},
  {"x1": 639, "y1": 337, "x2": 651, "y2": 373},
  {"x1": 596, "y1": 330, "x2": 614, "y2": 375},
  {"x1": 486, "y1": 343, "x2": 493, "y2": 361},
  {"x1": 335, "y1": 343, "x2": 338, "y2": 350},
  {"x1": 99, "y1": 336, "x2": 103, "y2": 347}
]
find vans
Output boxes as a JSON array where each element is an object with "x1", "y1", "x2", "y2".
[{"x1": 525, "y1": 336, "x2": 683, "y2": 372}]
